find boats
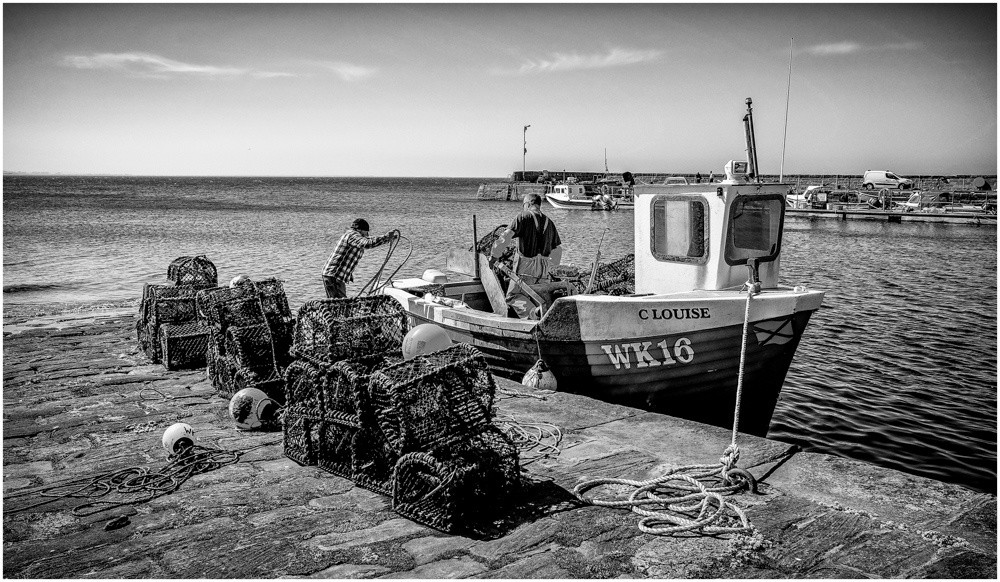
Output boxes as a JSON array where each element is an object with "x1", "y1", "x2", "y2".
[
  {"x1": 375, "y1": 35, "x2": 827, "y2": 437},
  {"x1": 784, "y1": 184, "x2": 997, "y2": 213},
  {"x1": 546, "y1": 176, "x2": 618, "y2": 210}
]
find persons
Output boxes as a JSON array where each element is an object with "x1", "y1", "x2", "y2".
[
  {"x1": 709, "y1": 171, "x2": 714, "y2": 182},
  {"x1": 484, "y1": 193, "x2": 562, "y2": 319},
  {"x1": 322, "y1": 218, "x2": 396, "y2": 297},
  {"x1": 696, "y1": 172, "x2": 702, "y2": 183}
]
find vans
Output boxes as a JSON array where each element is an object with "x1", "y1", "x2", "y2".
[{"x1": 863, "y1": 169, "x2": 913, "y2": 190}]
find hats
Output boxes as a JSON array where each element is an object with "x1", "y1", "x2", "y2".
[
  {"x1": 522, "y1": 194, "x2": 541, "y2": 206},
  {"x1": 351, "y1": 219, "x2": 369, "y2": 231}
]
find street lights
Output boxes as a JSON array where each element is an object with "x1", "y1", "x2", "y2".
[{"x1": 523, "y1": 124, "x2": 531, "y2": 180}]
